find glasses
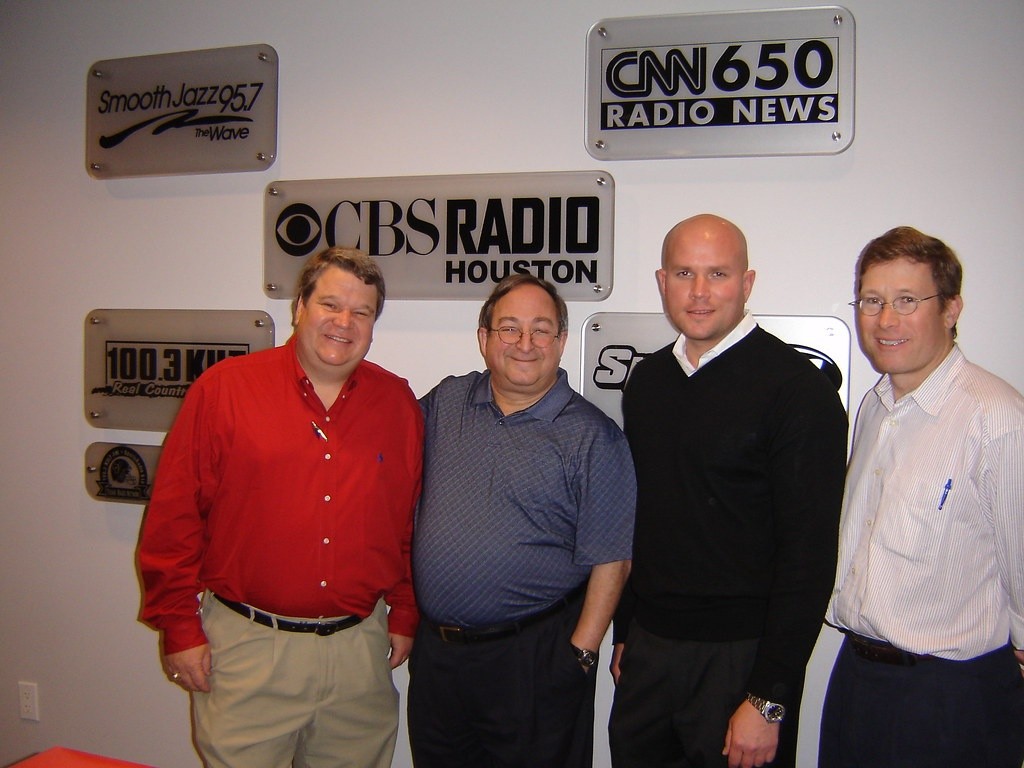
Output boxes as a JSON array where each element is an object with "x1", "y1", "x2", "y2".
[
  {"x1": 847, "y1": 292, "x2": 942, "y2": 316},
  {"x1": 488, "y1": 326, "x2": 561, "y2": 348}
]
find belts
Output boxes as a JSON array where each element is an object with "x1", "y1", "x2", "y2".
[
  {"x1": 419, "y1": 579, "x2": 586, "y2": 644},
  {"x1": 847, "y1": 630, "x2": 933, "y2": 667},
  {"x1": 209, "y1": 590, "x2": 363, "y2": 635}
]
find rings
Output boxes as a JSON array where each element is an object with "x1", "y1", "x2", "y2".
[{"x1": 172, "y1": 673, "x2": 178, "y2": 679}]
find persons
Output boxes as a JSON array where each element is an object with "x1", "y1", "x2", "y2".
[
  {"x1": 407, "y1": 274, "x2": 637, "y2": 768},
  {"x1": 609, "y1": 213, "x2": 849, "y2": 768},
  {"x1": 134, "y1": 246, "x2": 424, "y2": 768},
  {"x1": 819, "y1": 226, "x2": 1024, "y2": 768}
]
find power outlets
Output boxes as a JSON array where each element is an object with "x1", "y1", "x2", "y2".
[{"x1": 18, "y1": 681, "x2": 39, "y2": 720}]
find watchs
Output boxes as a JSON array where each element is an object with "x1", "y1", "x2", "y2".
[
  {"x1": 569, "y1": 642, "x2": 599, "y2": 667},
  {"x1": 746, "y1": 692, "x2": 785, "y2": 723}
]
